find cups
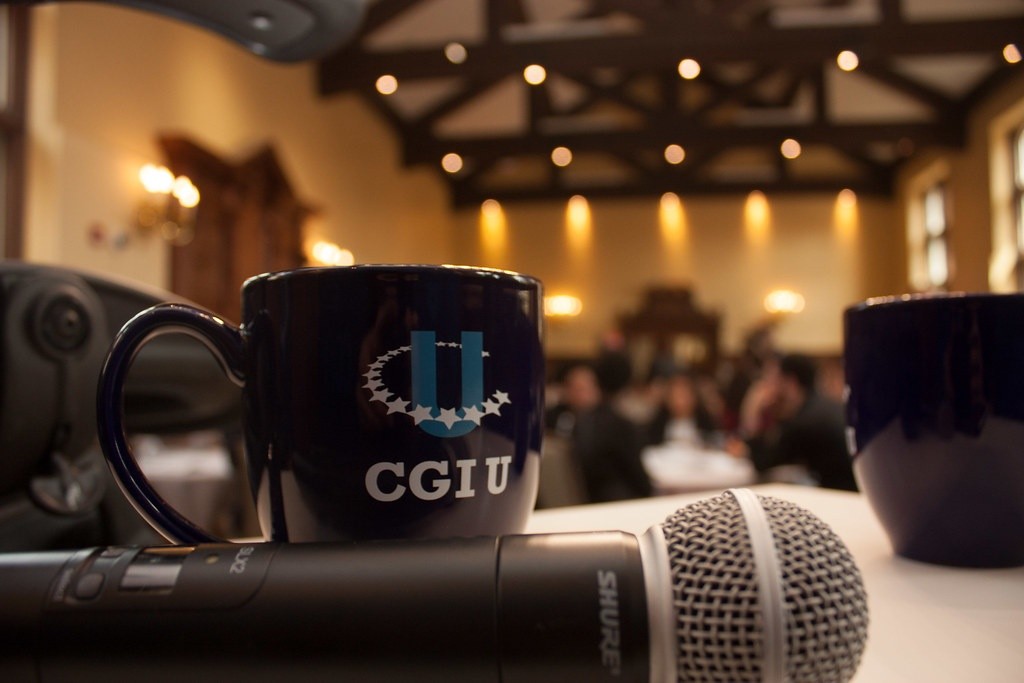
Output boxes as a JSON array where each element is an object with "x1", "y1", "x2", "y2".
[
  {"x1": 97, "y1": 265, "x2": 544, "y2": 546},
  {"x1": 844, "y1": 293, "x2": 1024, "y2": 567}
]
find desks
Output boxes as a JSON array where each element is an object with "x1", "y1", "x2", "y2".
[{"x1": 524, "y1": 482, "x2": 1024, "y2": 683}]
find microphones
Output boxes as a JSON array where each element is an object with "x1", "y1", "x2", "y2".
[{"x1": 0, "y1": 488, "x2": 868, "y2": 682}]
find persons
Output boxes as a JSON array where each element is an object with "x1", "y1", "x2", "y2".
[{"x1": 533, "y1": 348, "x2": 858, "y2": 508}]
